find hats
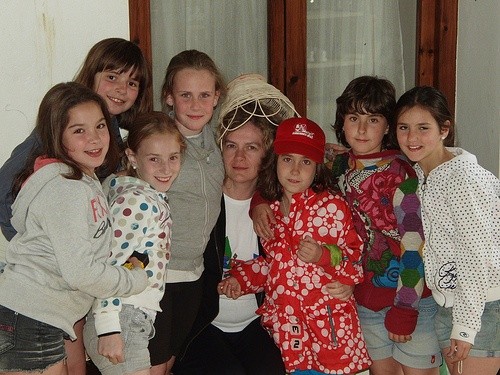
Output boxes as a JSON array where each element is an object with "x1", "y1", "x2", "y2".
[{"x1": 272, "y1": 117, "x2": 326, "y2": 164}]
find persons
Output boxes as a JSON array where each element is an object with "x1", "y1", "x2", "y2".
[
  {"x1": 217, "y1": 119, "x2": 365, "y2": 375},
  {"x1": 0, "y1": 38, "x2": 225, "y2": 375},
  {"x1": 174, "y1": 101, "x2": 357, "y2": 374},
  {"x1": 324, "y1": 87, "x2": 500, "y2": 375},
  {"x1": 250, "y1": 74, "x2": 443, "y2": 375}
]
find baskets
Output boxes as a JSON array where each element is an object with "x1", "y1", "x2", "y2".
[{"x1": 215, "y1": 73, "x2": 302, "y2": 151}]
